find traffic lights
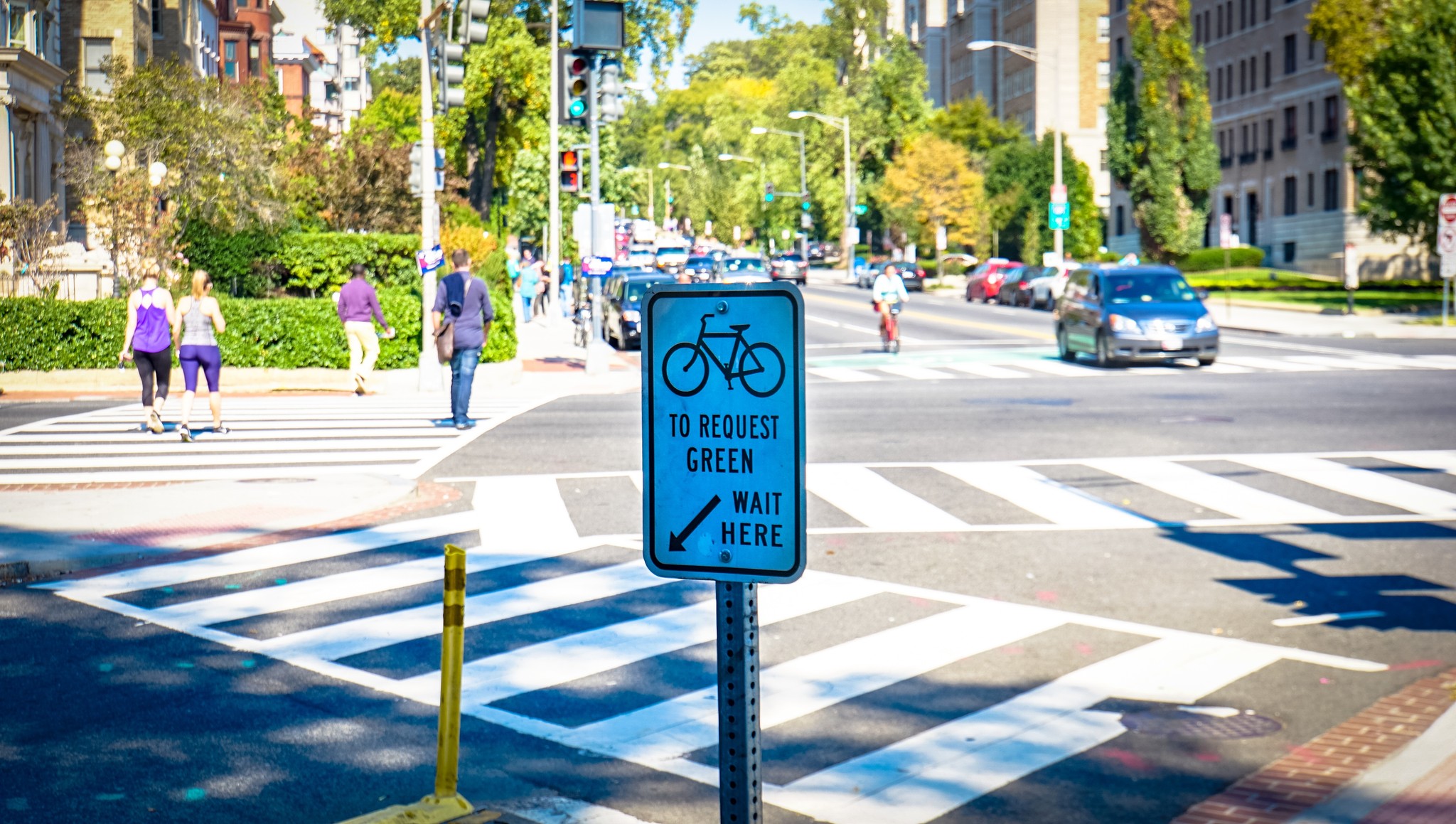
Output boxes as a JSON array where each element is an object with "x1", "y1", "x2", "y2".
[
  {"x1": 799, "y1": 191, "x2": 810, "y2": 213},
  {"x1": 573, "y1": 0, "x2": 622, "y2": 55},
  {"x1": 435, "y1": 42, "x2": 468, "y2": 114},
  {"x1": 557, "y1": 49, "x2": 591, "y2": 133},
  {"x1": 854, "y1": 206, "x2": 868, "y2": 216},
  {"x1": 447, "y1": 0, "x2": 493, "y2": 45},
  {"x1": 558, "y1": 150, "x2": 578, "y2": 193},
  {"x1": 764, "y1": 184, "x2": 774, "y2": 203}
]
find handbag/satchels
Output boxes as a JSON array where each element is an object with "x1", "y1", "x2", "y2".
[{"x1": 432, "y1": 322, "x2": 454, "y2": 364}]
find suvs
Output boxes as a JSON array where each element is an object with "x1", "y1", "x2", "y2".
[{"x1": 1053, "y1": 265, "x2": 1219, "y2": 368}]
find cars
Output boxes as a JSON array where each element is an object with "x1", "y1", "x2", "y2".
[
  {"x1": 854, "y1": 254, "x2": 978, "y2": 291},
  {"x1": 587, "y1": 218, "x2": 839, "y2": 349},
  {"x1": 964, "y1": 259, "x2": 1082, "y2": 312}
]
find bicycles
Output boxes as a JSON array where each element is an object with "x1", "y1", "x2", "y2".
[{"x1": 881, "y1": 300, "x2": 906, "y2": 352}]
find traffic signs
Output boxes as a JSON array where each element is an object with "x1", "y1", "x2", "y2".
[{"x1": 641, "y1": 281, "x2": 808, "y2": 583}]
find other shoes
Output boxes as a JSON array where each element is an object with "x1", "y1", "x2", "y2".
[
  {"x1": 179, "y1": 425, "x2": 195, "y2": 442},
  {"x1": 145, "y1": 426, "x2": 155, "y2": 434},
  {"x1": 354, "y1": 375, "x2": 368, "y2": 394},
  {"x1": 213, "y1": 424, "x2": 232, "y2": 435},
  {"x1": 150, "y1": 412, "x2": 165, "y2": 434},
  {"x1": 455, "y1": 417, "x2": 477, "y2": 429}
]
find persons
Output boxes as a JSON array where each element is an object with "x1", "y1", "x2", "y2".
[
  {"x1": 338, "y1": 264, "x2": 390, "y2": 396},
  {"x1": 517, "y1": 250, "x2": 550, "y2": 322},
  {"x1": 560, "y1": 257, "x2": 577, "y2": 317},
  {"x1": 873, "y1": 265, "x2": 909, "y2": 335},
  {"x1": 172, "y1": 269, "x2": 231, "y2": 443},
  {"x1": 119, "y1": 259, "x2": 176, "y2": 435},
  {"x1": 432, "y1": 248, "x2": 493, "y2": 430}
]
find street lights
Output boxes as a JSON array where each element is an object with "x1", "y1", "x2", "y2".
[
  {"x1": 148, "y1": 160, "x2": 168, "y2": 288},
  {"x1": 103, "y1": 140, "x2": 125, "y2": 298},
  {"x1": 623, "y1": 166, "x2": 655, "y2": 225},
  {"x1": 967, "y1": 40, "x2": 1064, "y2": 263},
  {"x1": 750, "y1": 126, "x2": 809, "y2": 267},
  {"x1": 788, "y1": 111, "x2": 850, "y2": 256}
]
